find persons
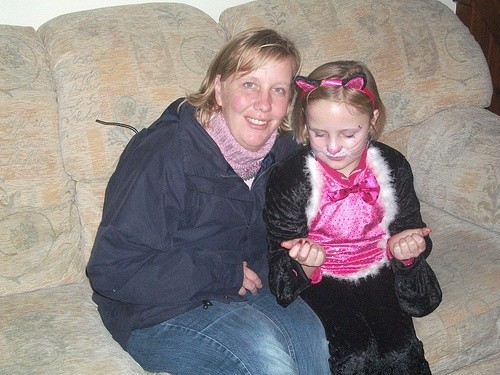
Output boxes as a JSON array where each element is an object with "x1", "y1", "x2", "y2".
[
  {"x1": 262, "y1": 61, "x2": 443, "y2": 375},
  {"x1": 85, "y1": 29, "x2": 331, "y2": 375}
]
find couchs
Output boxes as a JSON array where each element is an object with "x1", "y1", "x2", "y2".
[{"x1": 0, "y1": 3, "x2": 500, "y2": 375}]
